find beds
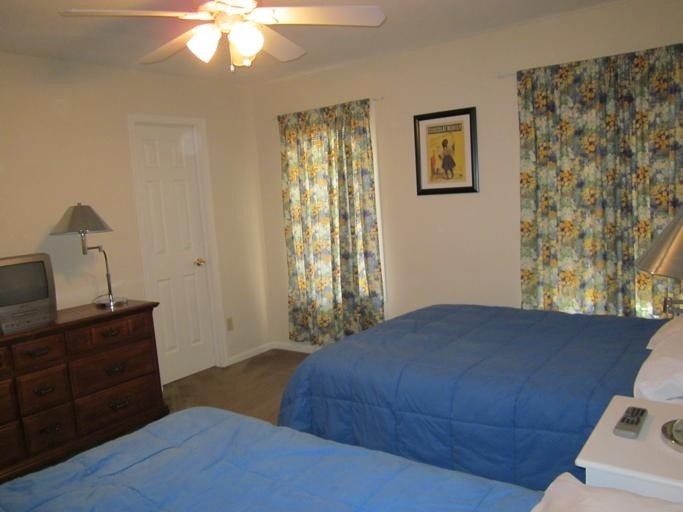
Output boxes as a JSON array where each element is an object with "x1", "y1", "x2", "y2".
[
  {"x1": 279, "y1": 303, "x2": 672, "y2": 495},
  {"x1": 0, "y1": 406, "x2": 683, "y2": 512}
]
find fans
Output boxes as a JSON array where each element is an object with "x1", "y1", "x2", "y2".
[{"x1": 59, "y1": 0, "x2": 386, "y2": 65}]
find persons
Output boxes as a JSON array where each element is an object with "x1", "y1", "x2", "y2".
[{"x1": 437, "y1": 137, "x2": 456, "y2": 179}]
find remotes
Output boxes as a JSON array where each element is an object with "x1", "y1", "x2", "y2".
[{"x1": 613, "y1": 406, "x2": 649, "y2": 439}]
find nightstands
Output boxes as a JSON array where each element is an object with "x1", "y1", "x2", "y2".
[{"x1": 575, "y1": 394, "x2": 683, "y2": 504}]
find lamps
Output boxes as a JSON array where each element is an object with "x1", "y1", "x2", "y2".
[
  {"x1": 635, "y1": 213, "x2": 683, "y2": 453},
  {"x1": 48, "y1": 202, "x2": 128, "y2": 308},
  {"x1": 184, "y1": 11, "x2": 264, "y2": 64}
]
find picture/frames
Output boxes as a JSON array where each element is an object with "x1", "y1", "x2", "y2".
[{"x1": 413, "y1": 106, "x2": 479, "y2": 195}]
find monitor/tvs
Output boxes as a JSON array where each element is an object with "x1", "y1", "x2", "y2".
[{"x1": 0, "y1": 253, "x2": 58, "y2": 337}]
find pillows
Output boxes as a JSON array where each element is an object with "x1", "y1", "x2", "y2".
[
  {"x1": 530, "y1": 471, "x2": 683, "y2": 512},
  {"x1": 633, "y1": 328, "x2": 683, "y2": 403},
  {"x1": 646, "y1": 314, "x2": 683, "y2": 351}
]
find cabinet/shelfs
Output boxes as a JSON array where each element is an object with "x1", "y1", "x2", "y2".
[{"x1": 0, "y1": 298, "x2": 169, "y2": 485}]
